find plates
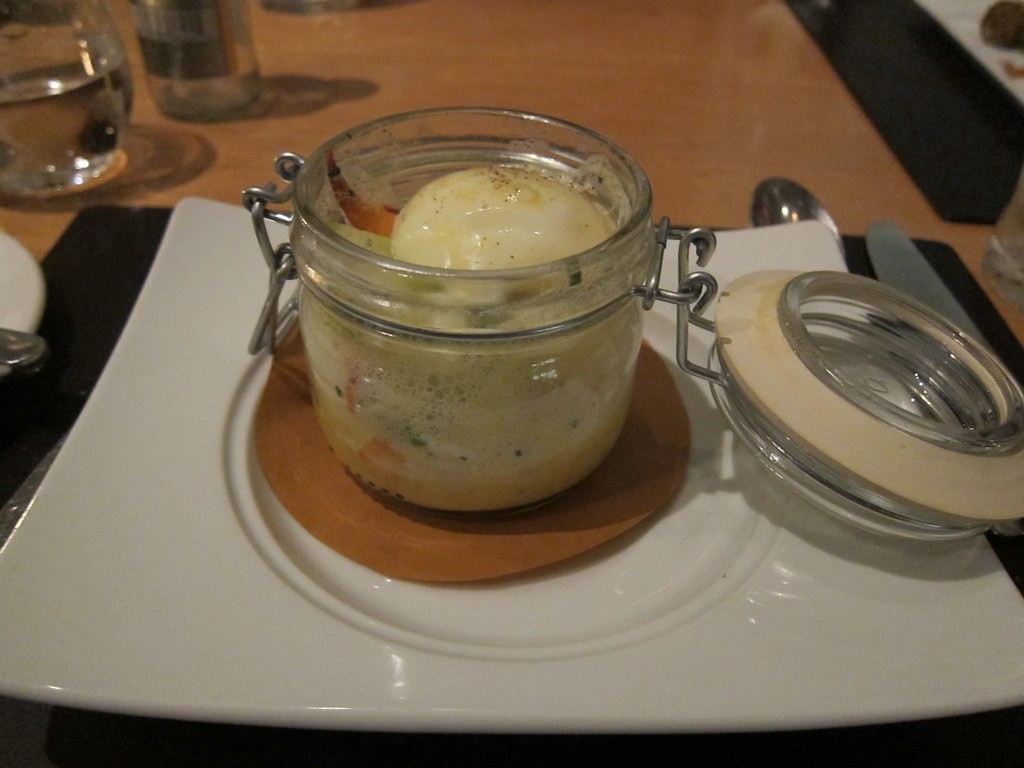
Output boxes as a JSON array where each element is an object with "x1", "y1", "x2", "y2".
[
  {"x1": 0, "y1": 227, "x2": 48, "y2": 380},
  {"x1": 1, "y1": 196, "x2": 1024, "y2": 735}
]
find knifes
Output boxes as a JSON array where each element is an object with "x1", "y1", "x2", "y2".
[{"x1": 865, "y1": 218, "x2": 998, "y2": 357}]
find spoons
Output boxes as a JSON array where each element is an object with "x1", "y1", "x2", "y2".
[{"x1": 750, "y1": 176, "x2": 847, "y2": 269}]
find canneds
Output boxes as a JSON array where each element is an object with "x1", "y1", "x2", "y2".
[{"x1": 239, "y1": 104, "x2": 1023, "y2": 544}]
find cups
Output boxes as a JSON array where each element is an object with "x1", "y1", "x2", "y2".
[
  {"x1": 240, "y1": 105, "x2": 703, "y2": 519},
  {"x1": 0, "y1": 0, "x2": 133, "y2": 204},
  {"x1": 128, "y1": 0, "x2": 263, "y2": 122}
]
